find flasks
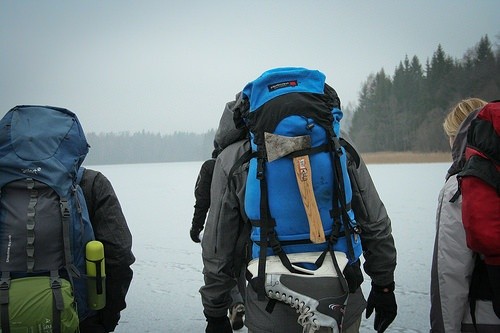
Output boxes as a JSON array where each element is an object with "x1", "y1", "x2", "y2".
[{"x1": 86, "y1": 241, "x2": 106, "y2": 310}]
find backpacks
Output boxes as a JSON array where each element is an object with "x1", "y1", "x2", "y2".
[
  {"x1": 444, "y1": 99, "x2": 500, "y2": 274},
  {"x1": 234, "y1": 67, "x2": 364, "y2": 264},
  {"x1": 0, "y1": 104, "x2": 116, "y2": 333}
]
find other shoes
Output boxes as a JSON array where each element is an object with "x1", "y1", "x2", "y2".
[{"x1": 229, "y1": 303, "x2": 245, "y2": 330}]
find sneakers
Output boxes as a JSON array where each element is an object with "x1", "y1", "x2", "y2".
[{"x1": 246, "y1": 252, "x2": 350, "y2": 332}]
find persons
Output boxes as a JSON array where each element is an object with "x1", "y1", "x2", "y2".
[
  {"x1": 428, "y1": 97, "x2": 500, "y2": 333},
  {"x1": 198, "y1": 73, "x2": 399, "y2": 333},
  {"x1": 190, "y1": 135, "x2": 224, "y2": 244},
  {"x1": 0, "y1": 103, "x2": 135, "y2": 332}
]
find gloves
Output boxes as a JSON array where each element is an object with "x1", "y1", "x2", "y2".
[
  {"x1": 366, "y1": 281, "x2": 398, "y2": 333},
  {"x1": 190, "y1": 224, "x2": 204, "y2": 243},
  {"x1": 205, "y1": 316, "x2": 233, "y2": 333}
]
